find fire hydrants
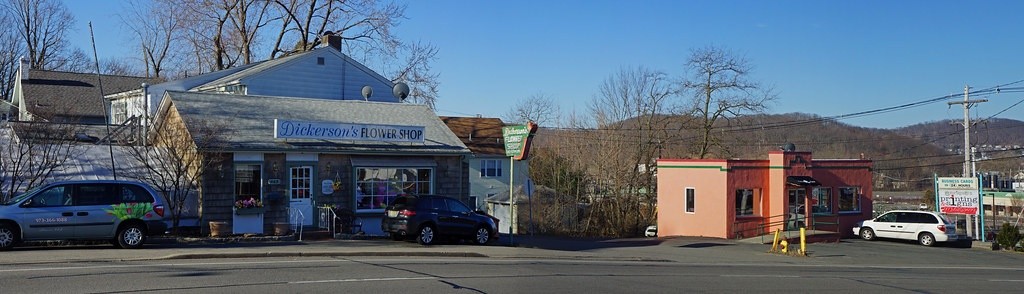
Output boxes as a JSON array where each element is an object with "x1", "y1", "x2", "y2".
[{"x1": 778, "y1": 235, "x2": 790, "y2": 252}]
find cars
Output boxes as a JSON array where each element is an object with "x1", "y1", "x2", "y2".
[
  {"x1": 381, "y1": 192, "x2": 499, "y2": 245},
  {"x1": 644, "y1": 225, "x2": 657, "y2": 237},
  {"x1": 918, "y1": 203, "x2": 927, "y2": 210}
]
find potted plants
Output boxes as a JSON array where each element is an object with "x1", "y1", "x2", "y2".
[{"x1": 333, "y1": 182, "x2": 344, "y2": 190}]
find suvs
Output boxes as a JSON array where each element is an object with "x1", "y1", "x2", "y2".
[
  {"x1": 0, "y1": 178, "x2": 174, "y2": 249},
  {"x1": 851, "y1": 209, "x2": 959, "y2": 247}
]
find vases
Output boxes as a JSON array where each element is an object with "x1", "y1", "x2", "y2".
[
  {"x1": 209, "y1": 222, "x2": 228, "y2": 237},
  {"x1": 272, "y1": 222, "x2": 289, "y2": 235},
  {"x1": 234, "y1": 206, "x2": 264, "y2": 214}
]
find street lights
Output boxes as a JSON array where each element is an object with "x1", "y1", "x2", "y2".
[{"x1": 988, "y1": 193, "x2": 995, "y2": 241}]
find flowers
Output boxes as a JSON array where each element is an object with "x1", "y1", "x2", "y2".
[{"x1": 235, "y1": 198, "x2": 262, "y2": 208}]
